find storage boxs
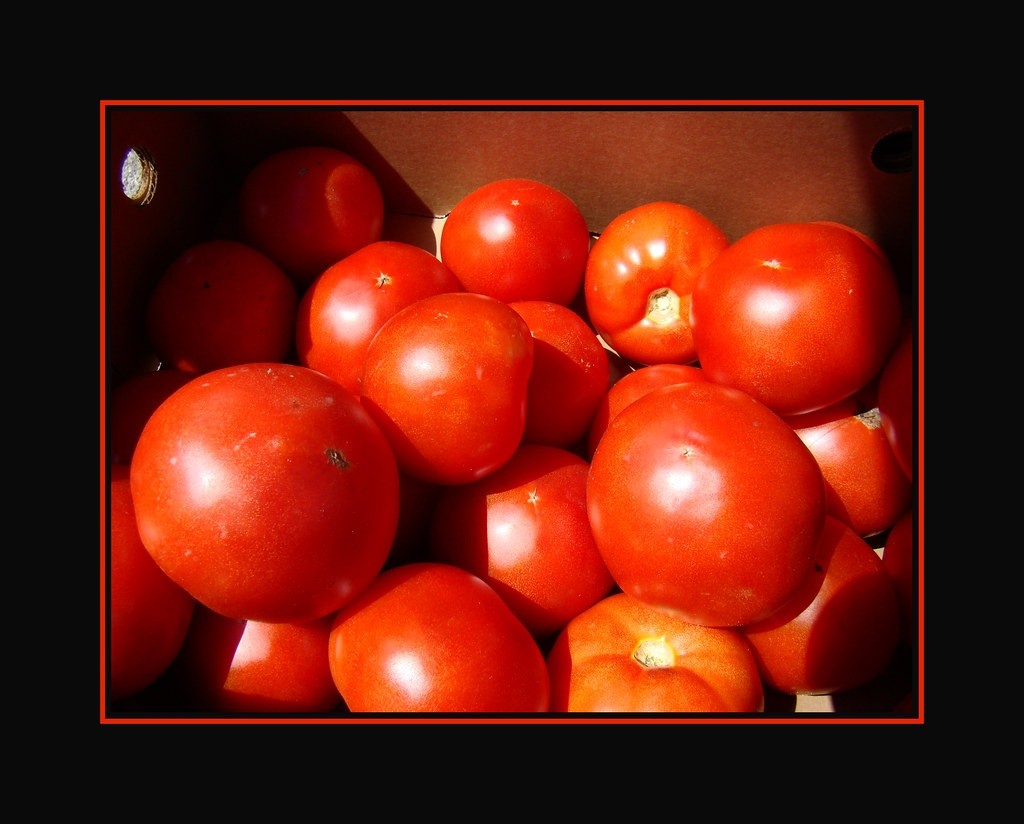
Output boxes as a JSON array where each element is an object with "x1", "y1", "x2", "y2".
[{"x1": 96, "y1": 99, "x2": 926, "y2": 726}]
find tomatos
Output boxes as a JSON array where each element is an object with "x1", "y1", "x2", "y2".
[{"x1": 110, "y1": 151, "x2": 913, "y2": 714}]
